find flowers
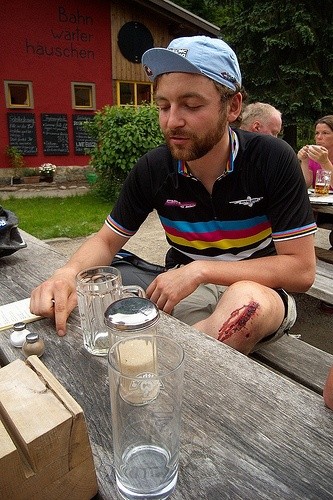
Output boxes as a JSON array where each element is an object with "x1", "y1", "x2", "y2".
[{"x1": 39, "y1": 163, "x2": 56, "y2": 175}]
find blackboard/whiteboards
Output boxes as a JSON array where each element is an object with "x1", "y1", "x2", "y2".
[
  {"x1": 72, "y1": 114, "x2": 99, "y2": 155},
  {"x1": 7, "y1": 112, "x2": 38, "y2": 155},
  {"x1": 41, "y1": 112, "x2": 69, "y2": 155}
]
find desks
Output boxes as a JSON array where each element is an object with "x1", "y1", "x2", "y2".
[
  {"x1": 305, "y1": 186, "x2": 333, "y2": 230},
  {"x1": 0, "y1": 228, "x2": 333, "y2": 500}
]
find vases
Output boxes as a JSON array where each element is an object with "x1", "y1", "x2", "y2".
[{"x1": 42, "y1": 175, "x2": 53, "y2": 183}]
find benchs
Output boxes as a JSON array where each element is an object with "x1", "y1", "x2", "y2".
[{"x1": 206, "y1": 226, "x2": 333, "y2": 396}]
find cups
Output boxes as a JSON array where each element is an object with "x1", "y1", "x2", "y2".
[
  {"x1": 75, "y1": 265, "x2": 146, "y2": 356},
  {"x1": 305, "y1": 145, "x2": 322, "y2": 153},
  {"x1": 107, "y1": 335, "x2": 185, "y2": 500},
  {"x1": 314, "y1": 169, "x2": 332, "y2": 196}
]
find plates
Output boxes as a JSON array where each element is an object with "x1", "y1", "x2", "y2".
[
  {"x1": 309, "y1": 196, "x2": 333, "y2": 203},
  {"x1": 308, "y1": 188, "x2": 333, "y2": 194}
]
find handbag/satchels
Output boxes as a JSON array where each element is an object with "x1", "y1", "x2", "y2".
[{"x1": 109, "y1": 248, "x2": 166, "y2": 297}]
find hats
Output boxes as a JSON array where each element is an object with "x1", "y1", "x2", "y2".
[{"x1": 142, "y1": 35, "x2": 241, "y2": 93}]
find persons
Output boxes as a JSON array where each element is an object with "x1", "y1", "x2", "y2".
[
  {"x1": 30, "y1": 37, "x2": 316, "y2": 358},
  {"x1": 238, "y1": 103, "x2": 333, "y2": 253}
]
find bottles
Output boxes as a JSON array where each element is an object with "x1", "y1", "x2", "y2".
[
  {"x1": 23, "y1": 333, "x2": 45, "y2": 358},
  {"x1": 103, "y1": 297, "x2": 161, "y2": 407},
  {"x1": 9, "y1": 323, "x2": 30, "y2": 347}
]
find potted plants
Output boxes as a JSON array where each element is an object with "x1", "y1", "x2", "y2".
[{"x1": 19, "y1": 167, "x2": 42, "y2": 184}]
